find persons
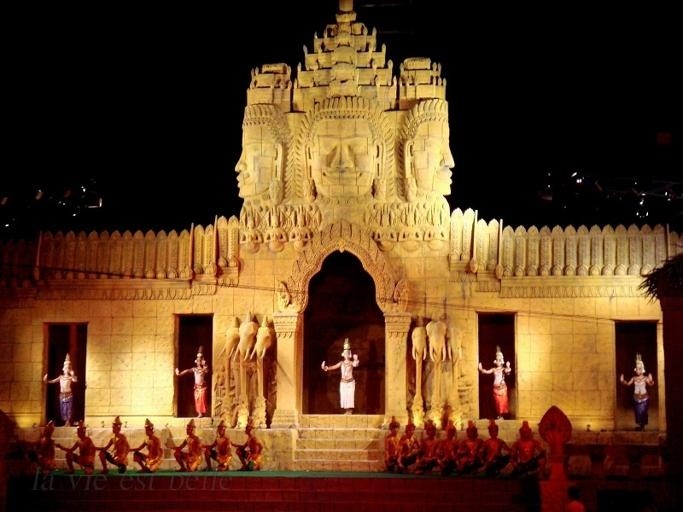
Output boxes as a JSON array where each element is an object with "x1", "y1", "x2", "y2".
[
  {"x1": 424, "y1": 211, "x2": 448, "y2": 241},
  {"x1": 238, "y1": 215, "x2": 261, "y2": 251},
  {"x1": 400, "y1": 213, "x2": 422, "y2": 242},
  {"x1": 289, "y1": 213, "x2": 312, "y2": 251},
  {"x1": 175, "y1": 344, "x2": 209, "y2": 418},
  {"x1": 292, "y1": 96, "x2": 388, "y2": 202},
  {"x1": 477, "y1": 345, "x2": 511, "y2": 421},
  {"x1": 30, "y1": 415, "x2": 264, "y2": 471},
  {"x1": 264, "y1": 214, "x2": 286, "y2": 252},
  {"x1": 395, "y1": 100, "x2": 454, "y2": 198},
  {"x1": 379, "y1": 414, "x2": 545, "y2": 479},
  {"x1": 620, "y1": 352, "x2": 654, "y2": 431},
  {"x1": 375, "y1": 213, "x2": 397, "y2": 245},
  {"x1": 320, "y1": 337, "x2": 359, "y2": 415},
  {"x1": 231, "y1": 106, "x2": 289, "y2": 209},
  {"x1": 40, "y1": 352, "x2": 77, "y2": 427}
]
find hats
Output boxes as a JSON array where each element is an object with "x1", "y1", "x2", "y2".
[
  {"x1": 44, "y1": 415, "x2": 257, "y2": 432},
  {"x1": 495, "y1": 345, "x2": 504, "y2": 358},
  {"x1": 342, "y1": 337, "x2": 353, "y2": 349},
  {"x1": 389, "y1": 415, "x2": 532, "y2": 432},
  {"x1": 62, "y1": 353, "x2": 72, "y2": 368},
  {"x1": 196, "y1": 345, "x2": 204, "y2": 357},
  {"x1": 635, "y1": 354, "x2": 645, "y2": 366}
]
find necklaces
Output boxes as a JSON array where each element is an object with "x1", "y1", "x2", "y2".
[{"x1": 495, "y1": 367, "x2": 502, "y2": 370}]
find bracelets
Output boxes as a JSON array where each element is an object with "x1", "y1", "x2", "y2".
[
  {"x1": 649, "y1": 381, "x2": 653, "y2": 384},
  {"x1": 324, "y1": 365, "x2": 328, "y2": 372},
  {"x1": 505, "y1": 368, "x2": 511, "y2": 373},
  {"x1": 203, "y1": 365, "x2": 208, "y2": 369},
  {"x1": 353, "y1": 360, "x2": 358, "y2": 363},
  {"x1": 622, "y1": 380, "x2": 627, "y2": 386}
]
